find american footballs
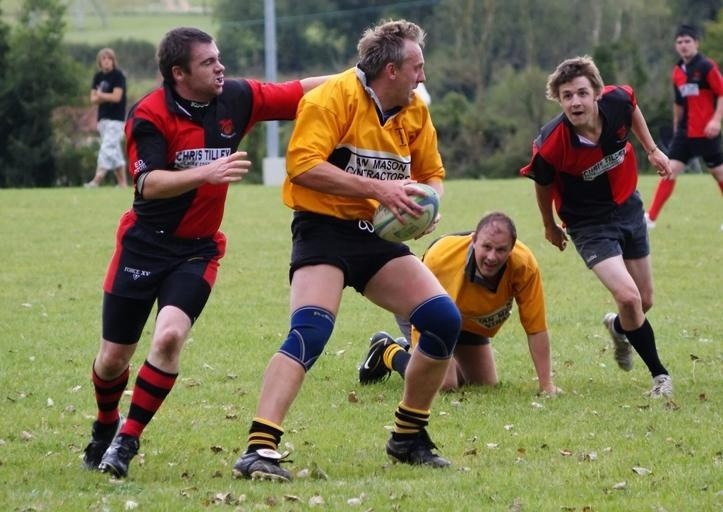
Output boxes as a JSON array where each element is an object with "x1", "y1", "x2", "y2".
[{"x1": 371, "y1": 181, "x2": 441, "y2": 244}]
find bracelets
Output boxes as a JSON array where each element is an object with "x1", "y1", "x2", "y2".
[{"x1": 645, "y1": 144, "x2": 658, "y2": 154}]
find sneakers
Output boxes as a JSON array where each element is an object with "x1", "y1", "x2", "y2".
[
  {"x1": 232, "y1": 448, "x2": 294, "y2": 484},
  {"x1": 652, "y1": 374, "x2": 672, "y2": 404},
  {"x1": 603, "y1": 313, "x2": 633, "y2": 372},
  {"x1": 359, "y1": 331, "x2": 408, "y2": 385},
  {"x1": 82, "y1": 416, "x2": 141, "y2": 480},
  {"x1": 386, "y1": 430, "x2": 450, "y2": 469}
]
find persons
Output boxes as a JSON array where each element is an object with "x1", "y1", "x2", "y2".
[
  {"x1": 80, "y1": 46, "x2": 131, "y2": 188},
  {"x1": 229, "y1": 17, "x2": 466, "y2": 481},
  {"x1": 79, "y1": 25, "x2": 344, "y2": 479},
  {"x1": 358, "y1": 213, "x2": 568, "y2": 400},
  {"x1": 644, "y1": 22, "x2": 722, "y2": 230},
  {"x1": 519, "y1": 54, "x2": 678, "y2": 405}
]
